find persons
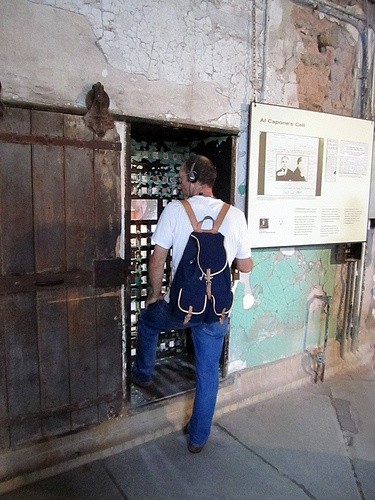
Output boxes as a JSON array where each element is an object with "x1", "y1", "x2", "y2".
[
  {"x1": 292, "y1": 157, "x2": 305, "y2": 181},
  {"x1": 276, "y1": 156, "x2": 294, "y2": 181},
  {"x1": 129, "y1": 154, "x2": 255, "y2": 454}
]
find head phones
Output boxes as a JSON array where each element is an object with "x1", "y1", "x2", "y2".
[{"x1": 188, "y1": 155, "x2": 201, "y2": 183}]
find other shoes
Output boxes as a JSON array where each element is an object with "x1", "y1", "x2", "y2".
[
  {"x1": 134, "y1": 372, "x2": 155, "y2": 390},
  {"x1": 184, "y1": 422, "x2": 203, "y2": 453}
]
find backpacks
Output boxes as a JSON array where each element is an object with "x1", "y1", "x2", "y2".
[{"x1": 170, "y1": 199, "x2": 233, "y2": 326}]
